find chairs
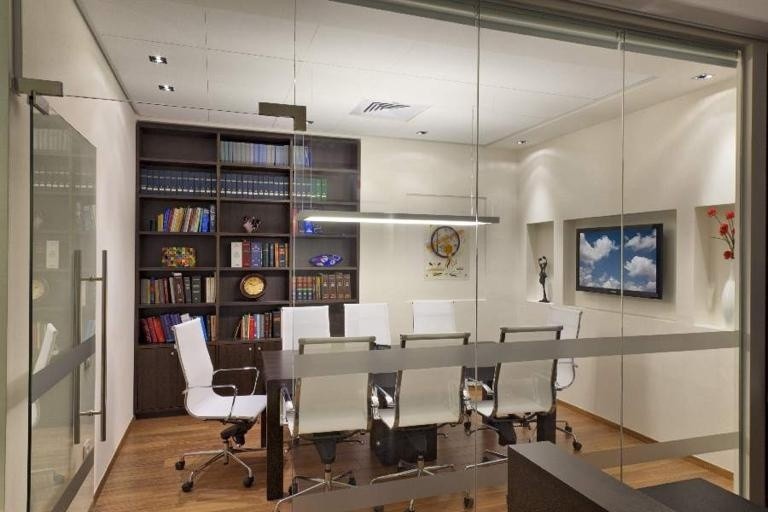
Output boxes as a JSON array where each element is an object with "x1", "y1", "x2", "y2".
[
  {"x1": 276, "y1": 306, "x2": 364, "y2": 451},
  {"x1": 464, "y1": 323, "x2": 566, "y2": 478},
  {"x1": 368, "y1": 334, "x2": 477, "y2": 511},
  {"x1": 520, "y1": 304, "x2": 582, "y2": 453},
  {"x1": 271, "y1": 328, "x2": 385, "y2": 511},
  {"x1": 162, "y1": 313, "x2": 265, "y2": 497},
  {"x1": 337, "y1": 300, "x2": 393, "y2": 438},
  {"x1": 407, "y1": 296, "x2": 473, "y2": 440}
]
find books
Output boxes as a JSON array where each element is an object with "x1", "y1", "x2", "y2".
[
  {"x1": 230, "y1": 239, "x2": 289, "y2": 267},
  {"x1": 138, "y1": 141, "x2": 328, "y2": 200},
  {"x1": 234, "y1": 311, "x2": 281, "y2": 340},
  {"x1": 296, "y1": 273, "x2": 352, "y2": 300},
  {"x1": 139, "y1": 204, "x2": 217, "y2": 341}
]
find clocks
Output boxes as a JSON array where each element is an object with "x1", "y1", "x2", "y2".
[
  {"x1": 31, "y1": 275, "x2": 51, "y2": 304},
  {"x1": 239, "y1": 273, "x2": 267, "y2": 299}
]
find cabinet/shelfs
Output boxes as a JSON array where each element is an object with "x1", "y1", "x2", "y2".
[
  {"x1": 291, "y1": 133, "x2": 358, "y2": 340},
  {"x1": 495, "y1": 443, "x2": 767, "y2": 511},
  {"x1": 217, "y1": 127, "x2": 290, "y2": 397},
  {"x1": 132, "y1": 119, "x2": 218, "y2": 421},
  {"x1": 32, "y1": 111, "x2": 98, "y2": 429}
]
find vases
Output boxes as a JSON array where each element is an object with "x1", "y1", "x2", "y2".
[{"x1": 719, "y1": 260, "x2": 735, "y2": 329}]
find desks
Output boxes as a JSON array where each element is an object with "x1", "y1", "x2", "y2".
[{"x1": 256, "y1": 339, "x2": 559, "y2": 501}]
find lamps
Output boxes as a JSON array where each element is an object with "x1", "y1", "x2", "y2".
[{"x1": 291, "y1": 67, "x2": 503, "y2": 228}]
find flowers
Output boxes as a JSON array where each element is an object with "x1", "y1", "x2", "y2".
[{"x1": 706, "y1": 207, "x2": 735, "y2": 262}]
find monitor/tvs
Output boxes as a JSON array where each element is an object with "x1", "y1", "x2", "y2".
[{"x1": 575, "y1": 224, "x2": 664, "y2": 300}]
